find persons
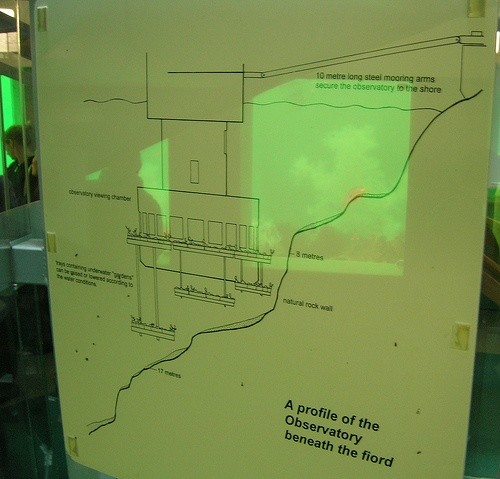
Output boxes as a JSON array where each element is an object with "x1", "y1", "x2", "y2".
[
  {"x1": 97, "y1": 148, "x2": 163, "y2": 243},
  {"x1": 0, "y1": 124, "x2": 41, "y2": 212}
]
